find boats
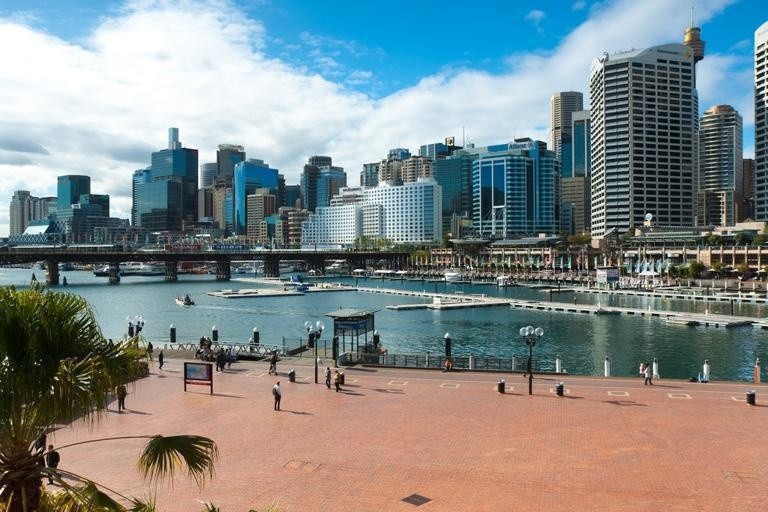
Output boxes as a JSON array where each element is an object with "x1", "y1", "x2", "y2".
[
  {"x1": 496, "y1": 275, "x2": 515, "y2": 288},
  {"x1": 326, "y1": 260, "x2": 350, "y2": 273},
  {"x1": 116, "y1": 260, "x2": 321, "y2": 277},
  {"x1": 92, "y1": 267, "x2": 122, "y2": 278},
  {"x1": 174, "y1": 296, "x2": 196, "y2": 308},
  {"x1": 440, "y1": 271, "x2": 462, "y2": 282}
]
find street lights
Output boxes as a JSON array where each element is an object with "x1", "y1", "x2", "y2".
[
  {"x1": 305, "y1": 320, "x2": 325, "y2": 384},
  {"x1": 517, "y1": 324, "x2": 545, "y2": 396},
  {"x1": 125, "y1": 314, "x2": 145, "y2": 363},
  {"x1": 231, "y1": 231, "x2": 237, "y2": 245}
]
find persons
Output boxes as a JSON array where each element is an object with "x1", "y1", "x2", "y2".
[
  {"x1": 194, "y1": 335, "x2": 233, "y2": 372},
  {"x1": 146, "y1": 342, "x2": 153, "y2": 361},
  {"x1": 46, "y1": 445, "x2": 61, "y2": 485},
  {"x1": 325, "y1": 367, "x2": 331, "y2": 389},
  {"x1": 640, "y1": 363, "x2": 646, "y2": 378},
  {"x1": 117, "y1": 384, "x2": 127, "y2": 413},
  {"x1": 334, "y1": 370, "x2": 342, "y2": 393},
  {"x1": 159, "y1": 350, "x2": 164, "y2": 371},
  {"x1": 108, "y1": 339, "x2": 114, "y2": 348},
  {"x1": 442, "y1": 359, "x2": 452, "y2": 373},
  {"x1": 35, "y1": 429, "x2": 46, "y2": 467},
  {"x1": 272, "y1": 381, "x2": 282, "y2": 411},
  {"x1": 644, "y1": 363, "x2": 653, "y2": 385},
  {"x1": 268, "y1": 351, "x2": 281, "y2": 376}
]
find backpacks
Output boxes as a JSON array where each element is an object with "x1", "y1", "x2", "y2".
[{"x1": 272, "y1": 388, "x2": 276, "y2": 394}]
